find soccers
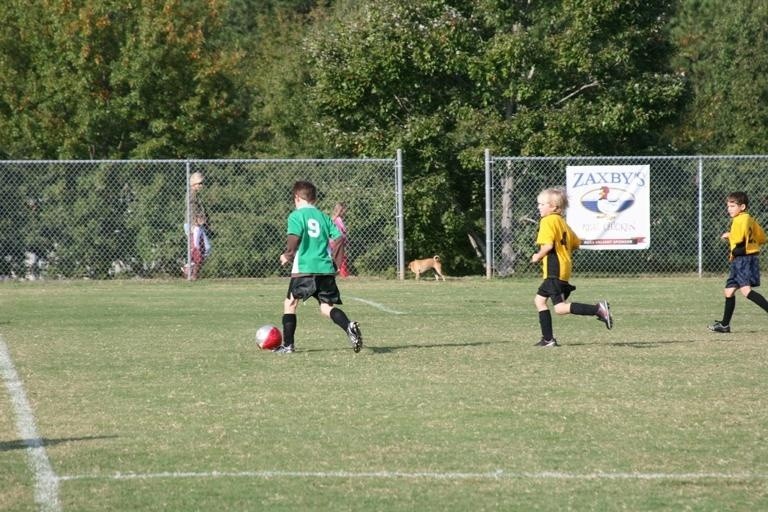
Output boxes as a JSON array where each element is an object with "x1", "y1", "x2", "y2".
[{"x1": 256, "y1": 324, "x2": 282, "y2": 349}]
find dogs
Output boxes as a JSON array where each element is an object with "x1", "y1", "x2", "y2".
[{"x1": 407, "y1": 255, "x2": 446, "y2": 282}]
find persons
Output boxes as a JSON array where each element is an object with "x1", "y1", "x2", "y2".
[
  {"x1": 181, "y1": 172, "x2": 212, "y2": 278},
  {"x1": 328, "y1": 201, "x2": 354, "y2": 278},
  {"x1": 272, "y1": 182, "x2": 362, "y2": 354},
  {"x1": 530, "y1": 187, "x2": 612, "y2": 347},
  {"x1": 706, "y1": 192, "x2": 768, "y2": 331}
]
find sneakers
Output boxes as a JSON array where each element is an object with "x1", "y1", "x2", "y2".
[
  {"x1": 708, "y1": 321, "x2": 730, "y2": 332},
  {"x1": 533, "y1": 338, "x2": 557, "y2": 347},
  {"x1": 346, "y1": 321, "x2": 362, "y2": 353},
  {"x1": 596, "y1": 300, "x2": 613, "y2": 329},
  {"x1": 272, "y1": 344, "x2": 294, "y2": 354}
]
